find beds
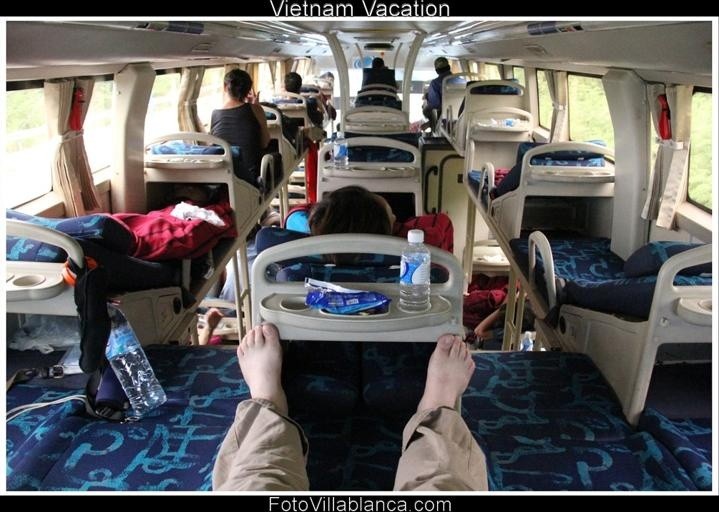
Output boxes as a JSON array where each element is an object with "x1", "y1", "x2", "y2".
[{"x1": 7, "y1": 81, "x2": 717, "y2": 493}]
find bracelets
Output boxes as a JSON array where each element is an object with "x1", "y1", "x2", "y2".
[{"x1": 497, "y1": 302, "x2": 506, "y2": 313}]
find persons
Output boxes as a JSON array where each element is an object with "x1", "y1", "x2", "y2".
[
  {"x1": 197, "y1": 267, "x2": 243, "y2": 316},
  {"x1": 261, "y1": 206, "x2": 278, "y2": 227},
  {"x1": 212, "y1": 324, "x2": 489, "y2": 490},
  {"x1": 308, "y1": 186, "x2": 396, "y2": 269},
  {"x1": 474, "y1": 289, "x2": 534, "y2": 341},
  {"x1": 211, "y1": 68, "x2": 271, "y2": 176},
  {"x1": 372, "y1": 58, "x2": 384, "y2": 68},
  {"x1": 284, "y1": 72, "x2": 325, "y2": 114},
  {"x1": 426, "y1": 57, "x2": 469, "y2": 107},
  {"x1": 189, "y1": 308, "x2": 224, "y2": 346},
  {"x1": 327, "y1": 122, "x2": 347, "y2": 159}
]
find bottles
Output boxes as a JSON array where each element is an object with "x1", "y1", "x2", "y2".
[
  {"x1": 332, "y1": 130, "x2": 350, "y2": 171},
  {"x1": 519, "y1": 331, "x2": 534, "y2": 352},
  {"x1": 101, "y1": 299, "x2": 169, "y2": 418},
  {"x1": 398, "y1": 229, "x2": 432, "y2": 313},
  {"x1": 497, "y1": 117, "x2": 521, "y2": 128}
]
